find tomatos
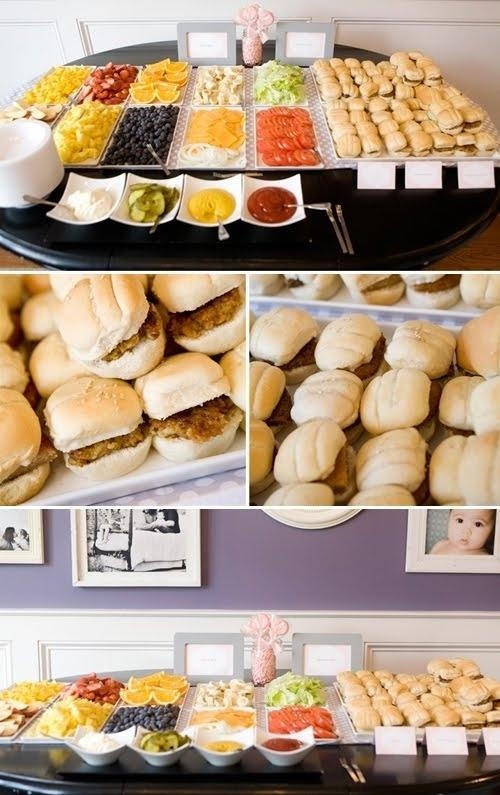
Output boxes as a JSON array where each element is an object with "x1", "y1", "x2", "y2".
[
  {"x1": 269, "y1": 705, "x2": 336, "y2": 739},
  {"x1": 258, "y1": 108, "x2": 319, "y2": 167}
]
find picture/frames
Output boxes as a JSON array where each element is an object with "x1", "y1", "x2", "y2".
[
  {"x1": 176, "y1": 18, "x2": 238, "y2": 66},
  {"x1": 173, "y1": 631, "x2": 245, "y2": 684},
  {"x1": 272, "y1": 20, "x2": 336, "y2": 66},
  {"x1": 0, "y1": 505, "x2": 47, "y2": 566},
  {"x1": 66, "y1": 509, "x2": 205, "y2": 589},
  {"x1": 402, "y1": 508, "x2": 499, "y2": 574},
  {"x1": 293, "y1": 631, "x2": 364, "y2": 681}
]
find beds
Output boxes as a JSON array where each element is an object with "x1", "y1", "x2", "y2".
[{"x1": 0, "y1": 38, "x2": 500, "y2": 271}]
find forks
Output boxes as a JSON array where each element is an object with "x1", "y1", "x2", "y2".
[{"x1": 337, "y1": 757, "x2": 358, "y2": 783}]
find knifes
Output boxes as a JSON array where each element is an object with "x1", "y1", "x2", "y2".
[{"x1": 352, "y1": 757, "x2": 366, "y2": 784}]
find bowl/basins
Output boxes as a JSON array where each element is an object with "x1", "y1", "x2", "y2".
[{"x1": 0, "y1": 117, "x2": 64, "y2": 209}]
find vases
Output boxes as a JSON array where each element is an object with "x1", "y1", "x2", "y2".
[
  {"x1": 241, "y1": 35, "x2": 264, "y2": 66},
  {"x1": 251, "y1": 639, "x2": 278, "y2": 684}
]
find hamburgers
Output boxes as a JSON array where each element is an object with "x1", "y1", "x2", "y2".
[
  {"x1": 312, "y1": 51, "x2": 498, "y2": 160},
  {"x1": 0, "y1": 273, "x2": 246, "y2": 506},
  {"x1": 250, "y1": 273, "x2": 499, "y2": 507},
  {"x1": 336, "y1": 659, "x2": 500, "y2": 732}
]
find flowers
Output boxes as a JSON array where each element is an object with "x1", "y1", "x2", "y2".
[
  {"x1": 239, "y1": 609, "x2": 288, "y2": 640},
  {"x1": 234, "y1": 5, "x2": 277, "y2": 34}
]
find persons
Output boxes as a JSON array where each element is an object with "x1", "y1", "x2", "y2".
[
  {"x1": 426, "y1": 508, "x2": 495, "y2": 556},
  {"x1": 0, "y1": 526, "x2": 31, "y2": 550},
  {"x1": 88, "y1": 508, "x2": 179, "y2": 548}
]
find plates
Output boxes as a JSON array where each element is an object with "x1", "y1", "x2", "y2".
[
  {"x1": 250, "y1": 313, "x2": 465, "y2": 504},
  {"x1": 48, "y1": 171, "x2": 311, "y2": 230},
  {"x1": 309, "y1": 65, "x2": 499, "y2": 164},
  {"x1": 65, "y1": 722, "x2": 316, "y2": 768},
  {"x1": 250, "y1": 276, "x2": 489, "y2": 316},
  {"x1": 1, "y1": 678, "x2": 339, "y2": 743},
  {"x1": 21, "y1": 63, "x2": 326, "y2": 171},
  {"x1": 16, "y1": 429, "x2": 246, "y2": 505}
]
